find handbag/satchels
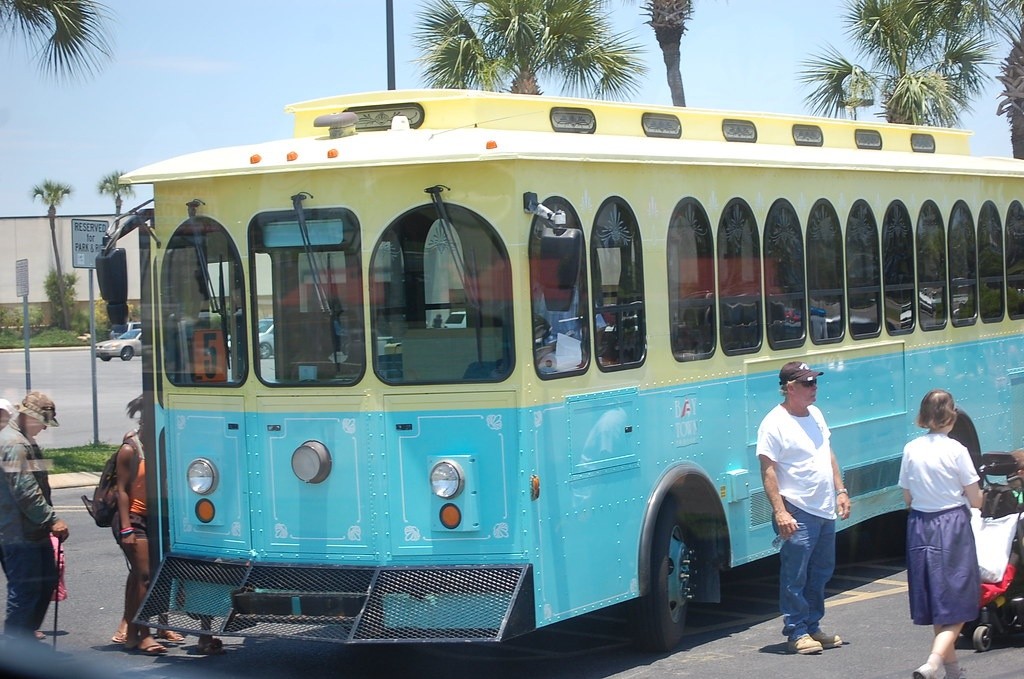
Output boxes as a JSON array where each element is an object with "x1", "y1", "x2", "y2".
[{"x1": 50, "y1": 532, "x2": 67, "y2": 601}]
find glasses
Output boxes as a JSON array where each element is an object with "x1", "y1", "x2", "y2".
[{"x1": 795, "y1": 379, "x2": 817, "y2": 386}]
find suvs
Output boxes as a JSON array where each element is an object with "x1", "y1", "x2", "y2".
[
  {"x1": 444, "y1": 310, "x2": 466, "y2": 328},
  {"x1": 228, "y1": 317, "x2": 275, "y2": 360},
  {"x1": 108, "y1": 321, "x2": 142, "y2": 339}
]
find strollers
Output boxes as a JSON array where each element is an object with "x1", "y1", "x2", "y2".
[{"x1": 958, "y1": 450, "x2": 1024, "y2": 651}]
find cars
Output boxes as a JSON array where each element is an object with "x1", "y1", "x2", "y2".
[{"x1": 95, "y1": 328, "x2": 143, "y2": 361}]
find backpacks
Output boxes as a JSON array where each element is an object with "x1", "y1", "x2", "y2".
[{"x1": 82, "y1": 438, "x2": 139, "y2": 527}]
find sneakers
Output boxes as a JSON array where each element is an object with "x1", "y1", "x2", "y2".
[
  {"x1": 787, "y1": 633, "x2": 823, "y2": 654},
  {"x1": 812, "y1": 632, "x2": 842, "y2": 648}
]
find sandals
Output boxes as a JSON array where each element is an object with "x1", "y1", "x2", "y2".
[
  {"x1": 138, "y1": 635, "x2": 166, "y2": 653},
  {"x1": 112, "y1": 622, "x2": 129, "y2": 643}
]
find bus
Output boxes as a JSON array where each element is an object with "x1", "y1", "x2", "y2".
[{"x1": 92, "y1": 87, "x2": 1024, "y2": 651}]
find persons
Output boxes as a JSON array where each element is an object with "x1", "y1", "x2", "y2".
[
  {"x1": 897, "y1": 388, "x2": 984, "y2": 679},
  {"x1": 468, "y1": 249, "x2": 607, "y2": 348},
  {"x1": 110, "y1": 395, "x2": 225, "y2": 655},
  {"x1": 0, "y1": 392, "x2": 70, "y2": 641},
  {"x1": 755, "y1": 361, "x2": 850, "y2": 654}
]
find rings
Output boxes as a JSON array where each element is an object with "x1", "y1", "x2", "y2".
[{"x1": 848, "y1": 511, "x2": 850, "y2": 513}]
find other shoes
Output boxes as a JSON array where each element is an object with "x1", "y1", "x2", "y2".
[
  {"x1": 198, "y1": 638, "x2": 226, "y2": 655},
  {"x1": 157, "y1": 628, "x2": 185, "y2": 641},
  {"x1": 914, "y1": 664, "x2": 936, "y2": 679},
  {"x1": 35, "y1": 630, "x2": 46, "y2": 639}
]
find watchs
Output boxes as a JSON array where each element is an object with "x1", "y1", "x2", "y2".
[{"x1": 836, "y1": 489, "x2": 848, "y2": 495}]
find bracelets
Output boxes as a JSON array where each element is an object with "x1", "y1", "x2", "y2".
[
  {"x1": 120, "y1": 527, "x2": 134, "y2": 532},
  {"x1": 121, "y1": 530, "x2": 133, "y2": 536}
]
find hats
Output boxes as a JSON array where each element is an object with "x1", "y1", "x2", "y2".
[
  {"x1": 0, "y1": 399, "x2": 14, "y2": 417},
  {"x1": 779, "y1": 361, "x2": 823, "y2": 385},
  {"x1": 16, "y1": 393, "x2": 60, "y2": 427}
]
